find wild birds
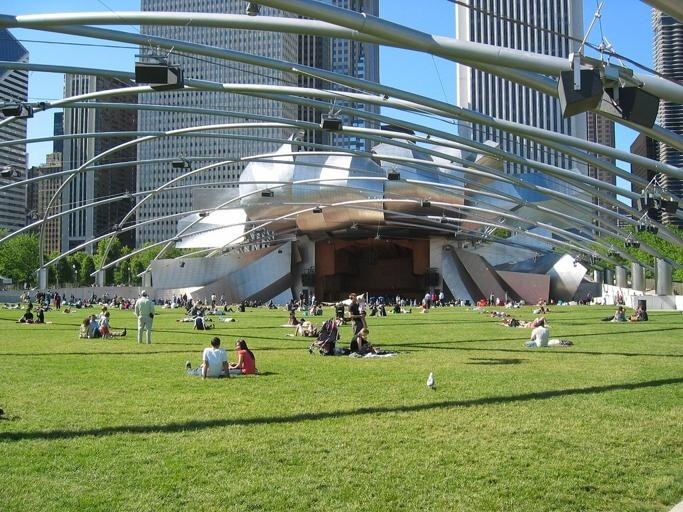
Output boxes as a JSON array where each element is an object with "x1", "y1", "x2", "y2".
[{"x1": 427, "y1": 372, "x2": 434, "y2": 388}]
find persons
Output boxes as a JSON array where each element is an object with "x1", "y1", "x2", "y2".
[
  {"x1": 135, "y1": 288, "x2": 155, "y2": 343},
  {"x1": 237, "y1": 299, "x2": 261, "y2": 312},
  {"x1": 477, "y1": 291, "x2": 511, "y2": 317},
  {"x1": 20, "y1": 288, "x2": 136, "y2": 313},
  {"x1": 163, "y1": 289, "x2": 235, "y2": 330},
  {"x1": 185, "y1": 337, "x2": 255, "y2": 380},
  {"x1": 614, "y1": 305, "x2": 647, "y2": 321},
  {"x1": 80, "y1": 306, "x2": 126, "y2": 340},
  {"x1": 370, "y1": 295, "x2": 406, "y2": 316},
  {"x1": 19, "y1": 308, "x2": 44, "y2": 324},
  {"x1": 152, "y1": 297, "x2": 164, "y2": 305},
  {"x1": 422, "y1": 289, "x2": 465, "y2": 309},
  {"x1": 14, "y1": 303, "x2": 21, "y2": 309},
  {"x1": 534, "y1": 298, "x2": 547, "y2": 314},
  {"x1": 269, "y1": 300, "x2": 277, "y2": 309},
  {"x1": 526, "y1": 317, "x2": 549, "y2": 347},
  {"x1": 286, "y1": 289, "x2": 375, "y2": 355}
]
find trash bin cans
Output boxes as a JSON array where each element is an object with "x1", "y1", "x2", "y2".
[
  {"x1": 638, "y1": 300, "x2": 646, "y2": 311},
  {"x1": 336, "y1": 305, "x2": 344, "y2": 318}
]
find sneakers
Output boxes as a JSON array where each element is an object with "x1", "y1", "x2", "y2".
[{"x1": 186, "y1": 361, "x2": 191, "y2": 369}]
